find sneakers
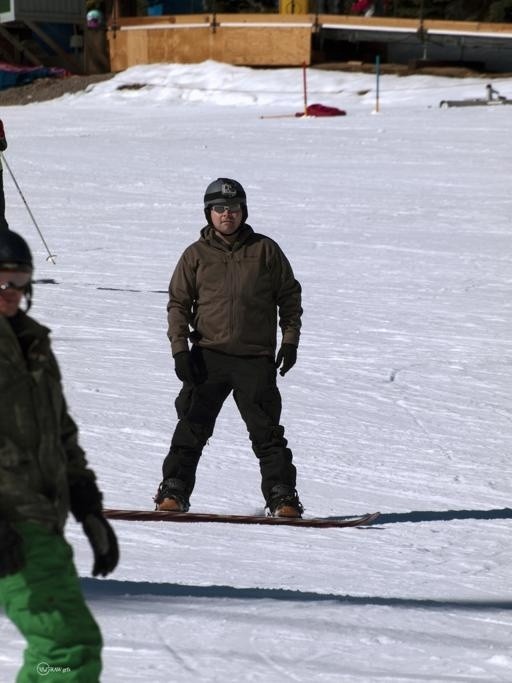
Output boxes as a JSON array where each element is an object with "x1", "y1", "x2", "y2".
[
  {"x1": 265, "y1": 484, "x2": 304, "y2": 519},
  {"x1": 154, "y1": 474, "x2": 191, "y2": 512}
]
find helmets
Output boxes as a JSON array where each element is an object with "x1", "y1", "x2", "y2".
[
  {"x1": 1, "y1": 228, "x2": 34, "y2": 264},
  {"x1": 203, "y1": 177, "x2": 247, "y2": 209}
]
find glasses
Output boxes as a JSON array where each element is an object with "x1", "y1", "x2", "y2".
[
  {"x1": 1, "y1": 265, "x2": 33, "y2": 290},
  {"x1": 214, "y1": 204, "x2": 241, "y2": 213}
]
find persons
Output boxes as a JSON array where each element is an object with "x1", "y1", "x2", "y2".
[
  {"x1": 153, "y1": 176, "x2": 305, "y2": 518},
  {"x1": 0, "y1": 230, "x2": 119, "y2": 683}
]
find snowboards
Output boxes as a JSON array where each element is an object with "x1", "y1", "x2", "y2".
[{"x1": 102, "y1": 507, "x2": 380, "y2": 526}]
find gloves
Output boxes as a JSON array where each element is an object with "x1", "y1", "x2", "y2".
[
  {"x1": 274, "y1": 342, "x2": 298, "y2": 376},
  {"x1": 175, "y1": 351, "x2": 200, "y2": 387},
  {"x1": 82, "y1": 509, "x2": 122, "y2": 578}
]
